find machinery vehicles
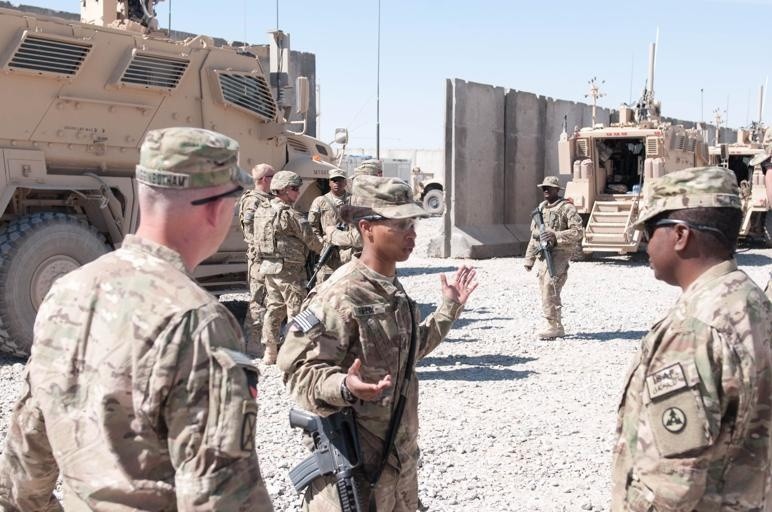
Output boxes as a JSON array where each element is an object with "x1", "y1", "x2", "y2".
[
  {"x1": 707, "y1": 73, "x2": 772, "y2": 250},
  {"x1": 405, "y1": 147, "x2": 445, "y2": 216},
  {"x1": 0, "y1": 0, "x2": 360, "y2": 361},
  {"x1": 549, "y1": 26, "x2": 716, "y2": 265}
]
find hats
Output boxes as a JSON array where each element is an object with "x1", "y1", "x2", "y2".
[
  {"x1": 328, "y1": 168, "x2": 348, "y2": 182},
  {"x1": 133, "y1": 125, "x2": 256, "y2": 191},
  {"x1": 533, "y1": 173, "x2": 568, "y2": 191},
  {"x1": 348, "y1": 171, "x2": 432, "y2": 221},
  {"x1": 347, "y1": 158, "x2": 384, "y2": 181},
  {"x1": 625, "y1": 160, "x2": 748, "y2": 234}
]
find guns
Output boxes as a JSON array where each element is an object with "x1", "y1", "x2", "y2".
[
  {"x1": 305, "y1": 227, "x2": 343, "y2": 287},
  {"x1": 289, "y1": 407, "x2": 377, "y2": 512},
  {"x1": 530, "y1": 208, "x2": 556, "y2": 278}
]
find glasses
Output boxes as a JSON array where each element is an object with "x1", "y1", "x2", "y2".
[
  {"x1": 352, "y1": 214, "x2": 417, "y2": 230},
  {"x1": 639, "y1": 215, "x2": 731, "y2": 244},
  {"x1": 190, "y1": 185, "x2": 247, "y2": 211},
  {"x1": 285, "y1": 187, "x2": 300, "y2": 191},
  {"x1": 259, "y1": 174, "x2": 273, "y2": 181}
]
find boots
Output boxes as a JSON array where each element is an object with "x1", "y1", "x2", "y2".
[{"x1": 537, "y1": 319, "x2": 567, "y2": 341}]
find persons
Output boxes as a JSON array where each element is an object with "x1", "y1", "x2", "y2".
[
  {"x1": 0, "y1": 126, "x2": 275, "y2": 512},
  {"x1": 746, "y1": 141, "x2": 772, "y2": 301},
  {"x1": 236, "y1": 161, "x2": 383, "y2": 365},
  {"x1": 275, "y1": 172, "x2": 480, "y2": 512},
  {"x1": 611, "y1": 167, "x2": 772, "y2": 512},
  {"x1": 523, "y1": 175, "x2": 583, "y2": 340}
]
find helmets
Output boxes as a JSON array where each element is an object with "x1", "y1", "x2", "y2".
[{"x1": 269, "y1": 170, "x2": 303, "y2": 191}]
